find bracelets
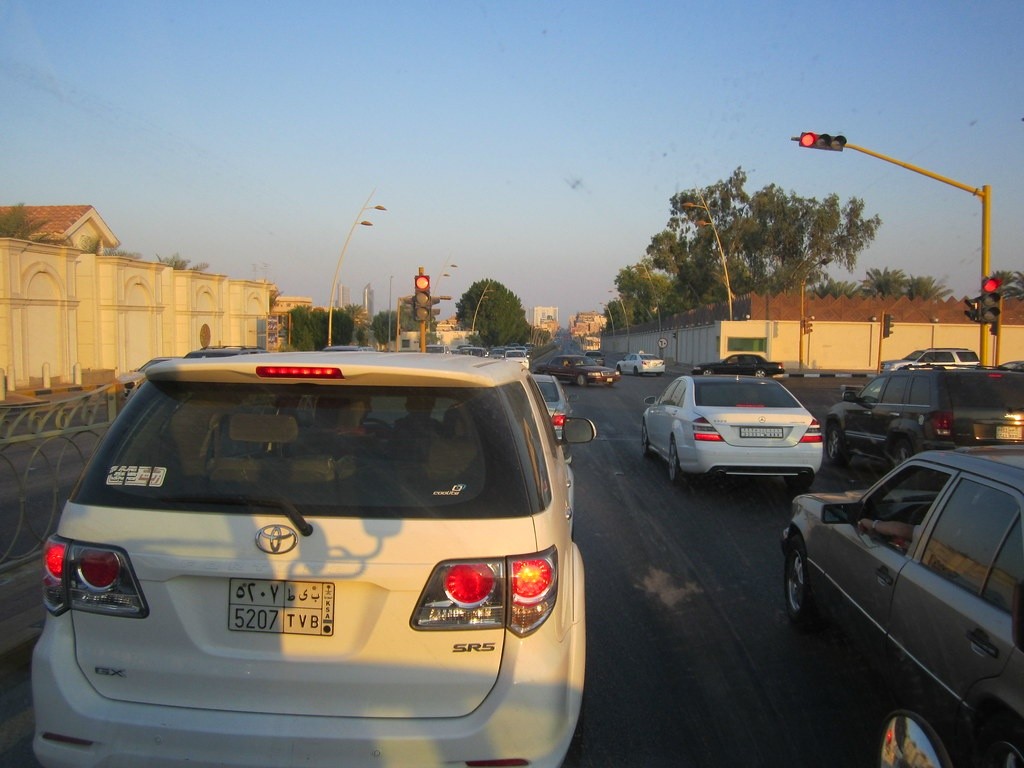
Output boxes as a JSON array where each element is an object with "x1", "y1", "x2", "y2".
[{"x1": 872, "y1": 520, "x2": 880, "y2": 532}]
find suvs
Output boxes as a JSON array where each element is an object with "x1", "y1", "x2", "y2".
[
  {"x1": 826, "y1": 364, "x2": 1024, "y2": 472},
  {"x1": 879, "y1": 347, "x2": 980, "y2": 372}
]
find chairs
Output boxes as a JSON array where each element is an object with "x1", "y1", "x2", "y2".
[
  {"x1": 336, "y1": 454, "x2": 483, "y2": 507},
  {"x1": 206, "y1": 412, "x2": 338, "y2": 508}
]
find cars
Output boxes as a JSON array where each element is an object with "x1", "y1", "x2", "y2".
[
  {"x1": 692, "y1": 353, "x2": 784, "y2": 378},
  {"x1": 616, "y1": 354, "x2": 666, "y2": 376},
  {"x1": 532, "y1": 374, "x2": 572, "y2": 440},
  {"x1": 533, "y1": 355, "x2": 621, "y2": 388},
  {"x1": 642, "y1": 374, "x2": 824, "y2": 489},
  {"x1": 586, "y1": 351, "x2": 606, "y2": 366},
  {"x1": 780, "y1": 446, "x2": 1024, "y2": 768},
  {"x1": 426, "y1": 343, "x2": 533, "y2": 370}
]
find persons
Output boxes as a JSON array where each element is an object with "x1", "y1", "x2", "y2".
[{"x1": 858, "y1": 517, "x2": 940, "y2": 542}]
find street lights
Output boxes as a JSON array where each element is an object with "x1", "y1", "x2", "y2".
[
  {"x1": 328, "y1": 189, "x2": 388, "y2": 345},
  {"x1": 471, "y1": 284, "x2": 493, "y2": 334},
  {"x1": 632, "y1": 263, "x2": 661, "y2": 339},
  {"x1": 682, "y1": 186, "x2": 732, "y2": 321},
  {"x1": 433, "y1": 262, "x2": 458, "y2": 296}
]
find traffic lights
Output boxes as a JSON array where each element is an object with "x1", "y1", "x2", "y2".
[
  {"x1": 884, "y1": 314, "x2": 893, "y2": 339},
  {"x1": 964, "y1": 297, "x2": 980, "y2": 322},
  {"x1": 804, "y1": 317, "x2": 812, "y2": 334},
  {"x1": 982, "y1": 277, "x2": 1002, "y2": 324},
  {"x1": 798, "y1": 131, "x2": 847, "y2": 152},
  {"x1": 415, "y1": 275, "x2": 431, "y2": 321},
  {"x1": 431, "y1": 296, "x2": 440, "y2": 317}
]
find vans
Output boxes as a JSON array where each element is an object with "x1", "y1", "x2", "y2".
[{"x1": 30, "y1": 352, "x2": 587, "y2": 768}]
